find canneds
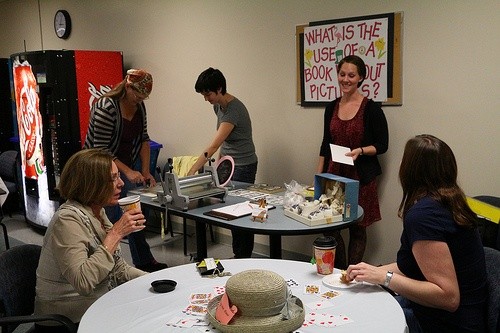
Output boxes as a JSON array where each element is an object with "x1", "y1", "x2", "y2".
[
  {"x1": 306, "y1": 187, "x2": 315, "y2": 197},
  {"x1": 12, "y1": 65, "x2": 45, "y2": 179}
]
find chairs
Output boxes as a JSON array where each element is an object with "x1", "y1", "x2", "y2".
[
  {"x1": 158, "y1": 156, "x2": 215, "y2": 244},
  {"x1": 483, "y1": 246, "x2": 500, "y2": 324},
  {"x1": 0, "y1": 243, "x2": 78, "y2": 333},
  {"x1": 0, "y1": 150, "x2": 19, "y2": 251}
]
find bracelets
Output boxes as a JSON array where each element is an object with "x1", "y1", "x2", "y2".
[
  {"x1": 384, "y1": 271, "x2": 393, "y2": 287},
  {"x1": 360, "y1": 147, "x2": 364, "y2": 155}
]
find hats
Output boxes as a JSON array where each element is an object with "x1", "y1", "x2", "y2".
[{"x1": 207, "y1": 270, "x2": 304, "y2": 333}]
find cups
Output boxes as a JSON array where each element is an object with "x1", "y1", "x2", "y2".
[
  {"x1": 312, "y1": 235, "x2": 337, "y2": 276},
  {"x1": 118, "y1": 195, "x2": 144, "y2": 233}
]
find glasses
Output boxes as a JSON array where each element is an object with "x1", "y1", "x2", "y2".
[{"x1": 131, "y1": 86, "x2": 150, "y2": 101}]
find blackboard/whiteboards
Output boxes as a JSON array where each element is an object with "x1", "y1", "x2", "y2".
[{"x1": 304, "y1": 18, "x2": 388, "y2": 105}]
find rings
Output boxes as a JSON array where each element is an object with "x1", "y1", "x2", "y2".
[{"x1": 136, "y1": 221, "x2": 138, "y2": 225}]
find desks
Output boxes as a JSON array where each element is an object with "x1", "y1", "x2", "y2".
[
  {"x1": 77, "y1": 257, "x2": 408, "y2": 333},
  {"x1": 125, "y1": 180, "x2": 365, "y2": 270}
]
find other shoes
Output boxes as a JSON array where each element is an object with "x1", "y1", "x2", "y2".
[{"x1": 135, "y1": 259, "x2": 168, "y2": 273}]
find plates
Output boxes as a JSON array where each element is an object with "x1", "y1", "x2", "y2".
[{"x1": 323, "y1": 274, "x2": 360, "y2": 289}]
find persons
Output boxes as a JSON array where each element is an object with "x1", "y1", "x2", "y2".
[
  {"x1": 318, "y1": 55, "x2": 390, "y2": 271},
  {"x1": 33, "y1": 148, "x2": 146, "y2": 333},
  {"x1": 83, "y1": 68, "x2": 168, "y2": 273},
  {"x1": 187, "y1": 68, "x2": 258, "y2": 258},
  {"x1": 347, "y1": 134, "x2": 491, "y2": 333}
]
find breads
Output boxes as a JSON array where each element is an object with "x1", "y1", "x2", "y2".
[{"x1": 339, "y1": 271, "x2": 354, "y2": 283}]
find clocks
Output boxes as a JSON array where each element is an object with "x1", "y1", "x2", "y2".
[{"x1": 53, "y1": 9, "x2": 71, "y2": 39}]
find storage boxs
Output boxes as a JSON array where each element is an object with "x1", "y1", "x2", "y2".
[{"x1": 314, "y1": 172, "x2": 359, "y2": 221}]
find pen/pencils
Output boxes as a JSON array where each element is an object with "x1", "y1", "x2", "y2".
[{"x1": 248, "y1": 203, "x2": 254, "y2": 209}]
